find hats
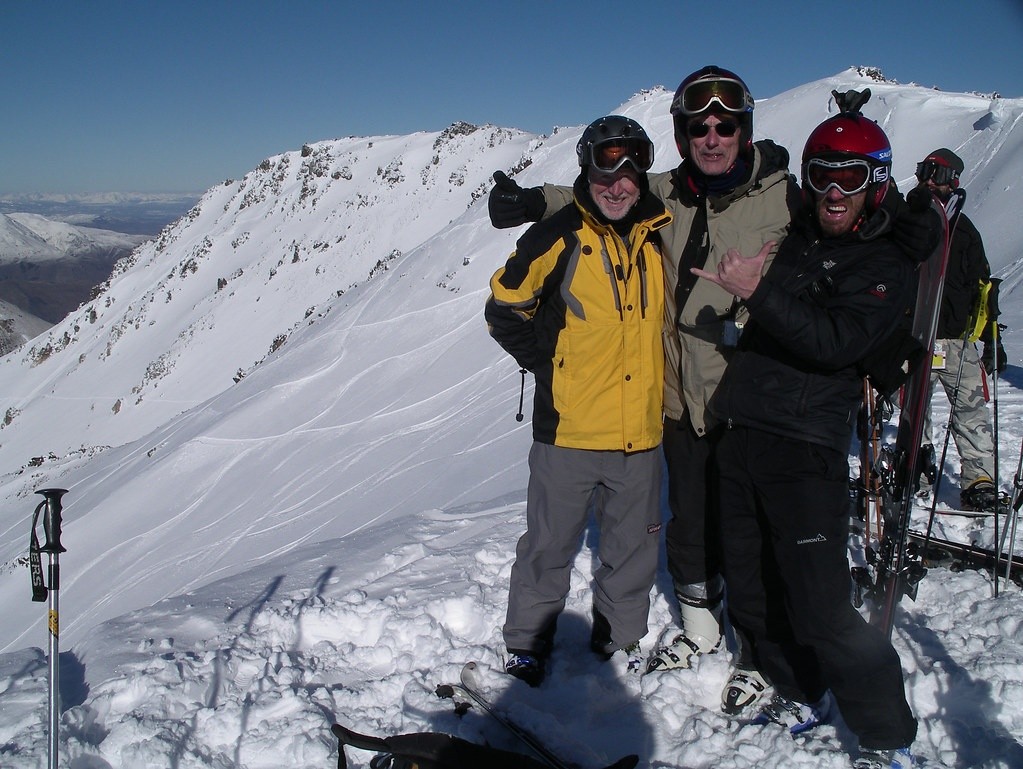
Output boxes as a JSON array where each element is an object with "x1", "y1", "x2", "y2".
[{"x1": 922, "y1": 148, "x2": 964, "y2": 186}]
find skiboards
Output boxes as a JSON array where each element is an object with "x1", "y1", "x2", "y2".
[
  {"x1": 847, "y1": 516, "x2": 1023, "y2": 582},
  {"x1": 864, "y1": 189, "x2": 967, "y2": 641}
]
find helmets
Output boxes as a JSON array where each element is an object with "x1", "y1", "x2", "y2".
[
  {"x1": 670, "y1": 65, "x2": 756, "y2": 160},
  {"x1": 576, "y1": 115, "x2": 654, "y2": 175},
  {"x1": 799, "y1": 114, "x2": 892, "y2": 207}
]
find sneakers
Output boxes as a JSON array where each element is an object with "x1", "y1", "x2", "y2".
[
  {"x1": 855, "y1": 747, "x2": 916, "y2": 768},
  {"x1": 722, "y1": 658, "x2": 774, "y2": 714},
  {"x1": 594, "y1": 641, "x2": 644, "y2": 674},
  {"x1": 754, "y1": 694, "x2": 833, "y2": 734},
  {"x1": 960, "y1": 481, "x2": 1011, "y2": 514},
  {"x1": 505, "y1": 650, "x2": 550, "y2": 685}
]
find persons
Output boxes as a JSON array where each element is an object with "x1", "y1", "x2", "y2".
[
  {"x1": 488, "y1": 64, "x2": 943, "y2": 715},
  {"x1": 689, "y1": 90, "x2": 942, "y2": 769},
  {"x1": 483, "y1": 115, "x2": 674, "y2": 687},
  {"x1": 898, "y1": 150, "x2": 1010, "y2": 514}
]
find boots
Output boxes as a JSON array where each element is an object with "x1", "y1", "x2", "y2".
[{"x1": 648, "y1": 591, "x2": 730, "y2": 671}]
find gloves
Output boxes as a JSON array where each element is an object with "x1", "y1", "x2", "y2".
[
  {"x1": 981, "y1": 344, "x2": 1007, "y2": 376},
  {"x1": 488, "y1": 170, "x2": 547, "y2": 229}
]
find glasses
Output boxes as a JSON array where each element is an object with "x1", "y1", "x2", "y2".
[
  {"x1": 806, "y1": 158, "x2": 872, "y2": 196},
  {"x1": 689, "y1": 122, "x2": 738, "y2": 139},
  {"x1": 678, "y1": 76, "x2": 750, "y2": 114},
  {"x1": 589, "y1": 136, "x2": 656, "y2": 174}
]
